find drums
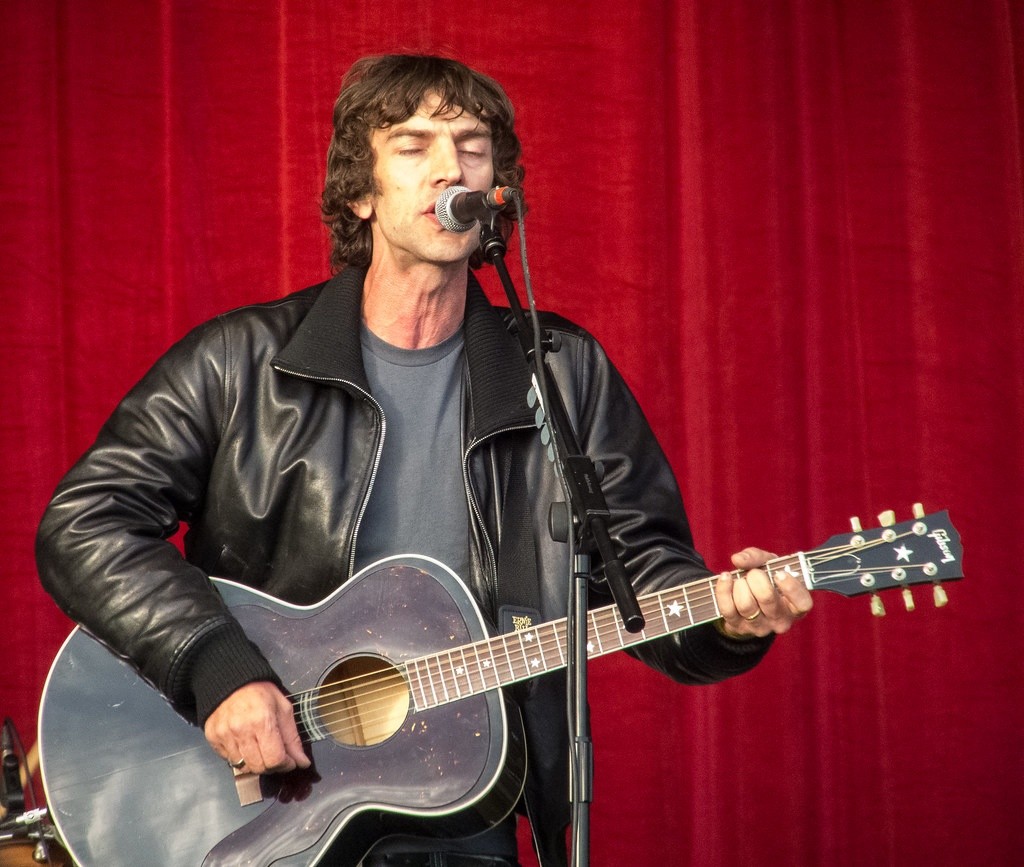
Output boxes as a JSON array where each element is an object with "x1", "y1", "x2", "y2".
[{"x1": 0, "y1": 832, "x2": 73, "y2": 867}]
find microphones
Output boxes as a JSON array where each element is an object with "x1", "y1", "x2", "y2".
[
  {"x1": 435, "y1": 185, "x2": 521, "y2": 234},
  {"x1": 2, "y1": 724, "x2": 13, "y2": 761}
]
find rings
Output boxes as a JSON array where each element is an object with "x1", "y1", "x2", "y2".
[
  {"x1": 232, "y1": 758, "x2": 245, "y2": 769},
  {"x1": 743, "y1": 609, "x2": 761, "y2": 621}
]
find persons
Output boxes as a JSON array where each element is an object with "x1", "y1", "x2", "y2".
[{"x1": 34, "y1": 55, "x2": 814, "y2": 867}]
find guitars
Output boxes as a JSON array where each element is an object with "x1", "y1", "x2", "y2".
[{"x1": 31, "y1": 501, "x2": 966, "y2": 865}]
